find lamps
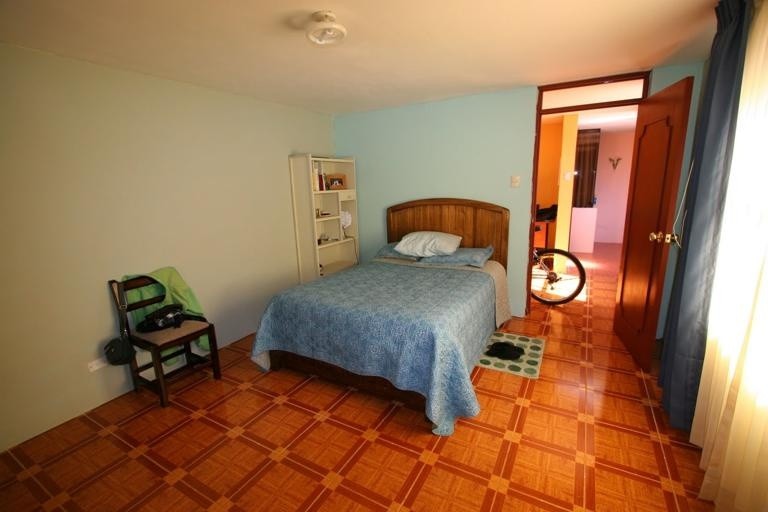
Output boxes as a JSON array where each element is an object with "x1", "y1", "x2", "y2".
[
  {"x1": 307, "y1": 12, "x2": 347, "y2": 46},
  {"x1": 340, "y1": 211, "x2": 352, "y2": 240}
]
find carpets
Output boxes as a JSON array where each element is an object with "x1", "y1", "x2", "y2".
[{"x1": 478, "y1": 325, "x2": 547, "y2": 380}]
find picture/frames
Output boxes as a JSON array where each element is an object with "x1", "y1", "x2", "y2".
[{"x1": 326, "y1": 174, "x2": 346, "y2": 189}]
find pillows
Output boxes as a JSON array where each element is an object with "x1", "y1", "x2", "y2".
[
  {"x1": 415, "y1": 245, "x2": 495, "y2": 269},
  {"x1": 374, "y1": 242, "x2": 418, "y2": 261},
  {"x1": 393, "y1": 230, "x2": 463, "y2": 255}
]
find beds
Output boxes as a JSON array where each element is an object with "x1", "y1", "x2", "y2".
[{"x1": 251, "y1": 195, "x2": 519, "y2": 436}]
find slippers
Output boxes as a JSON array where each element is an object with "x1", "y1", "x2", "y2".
[{"x1": 487, "y1": 342, "x2": 524, "y2": 359}]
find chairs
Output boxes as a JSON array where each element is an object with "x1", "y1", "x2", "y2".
[{"x1": 107, "y1": 267, "x2": 222, "y2": 406}]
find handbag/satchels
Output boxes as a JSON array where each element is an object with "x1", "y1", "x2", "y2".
[{"x1": 104, "y1": 334, "x2": 136, "y2": 365}]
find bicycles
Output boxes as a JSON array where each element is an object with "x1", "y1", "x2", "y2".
[{"x1": 530, "y1": 225, "x2": 588, "y2": 308}]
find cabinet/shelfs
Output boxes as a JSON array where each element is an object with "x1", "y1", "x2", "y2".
[
  {"x1": 289, "y1": 151, "x2": 361, "y2": 284},
  {"x1": 533, "y1": 221, "x2": 547, "y2": 249}
]
